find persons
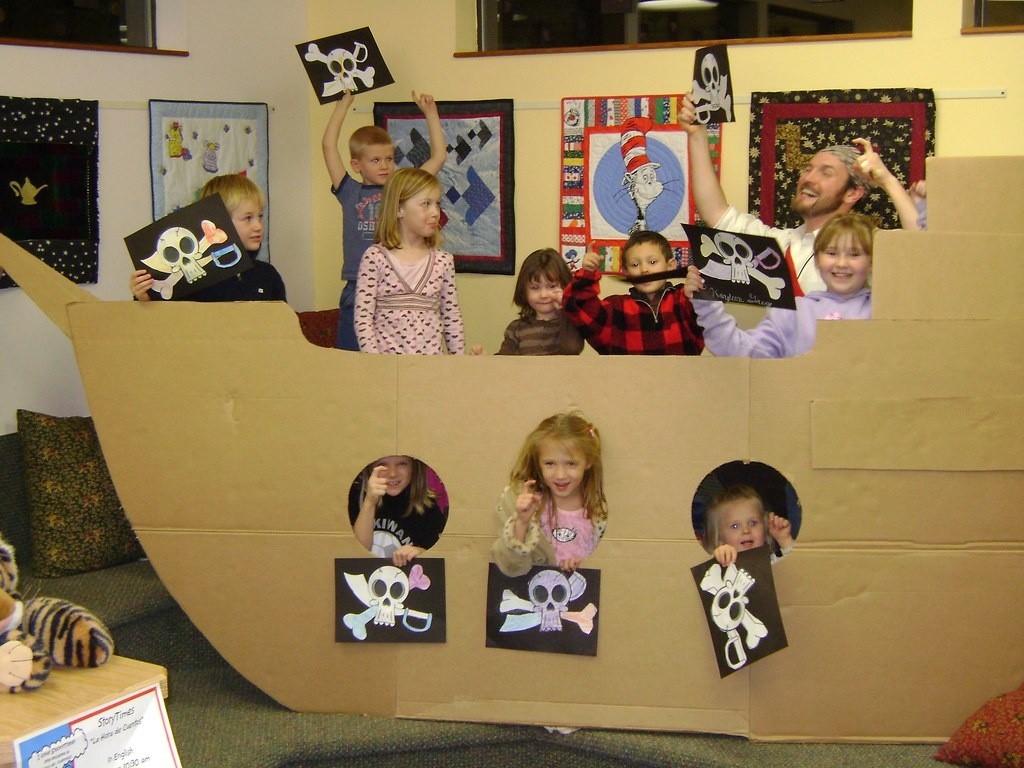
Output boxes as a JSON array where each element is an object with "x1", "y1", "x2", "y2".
[
  {"x1": 703, "y1": 485, "x2": 794, "y2": 567},
  {"x1": 490, "y1": 413, "x2": 607, "y2": 577},
  {"x1": 354, "y1": 167, "x2": 465, "y2": 354},
  {"x1": 471, "y1": 247, "x2": 585, "y2": 355},
  {"x1": 677, "y1": 92, "x2": 919, "y2": 297},
  {"x1": 129, "y1": 174, "x2": 287, "y2": 304},
  {"x1": 562, "y1": 231, "x2": 705, "y2": 356},
  {"x1": 322, "y1": 89, "x2": 446, "y2": 350},
  {"x1": 348, "y1": 455, "x2": 445, "y2": 567},
  {"x1": 684, "y1": 180, "x2": 927, "y2": 359}
]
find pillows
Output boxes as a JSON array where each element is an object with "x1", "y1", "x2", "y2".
[
  {"x1": 17, "y1": 408, "x2": 140, "y2": 580},
  {"x1": 933, "y1": 681, "x2": 1024, "y2": 768}
]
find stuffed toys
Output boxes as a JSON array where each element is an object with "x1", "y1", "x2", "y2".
[{"x1": 0, "y1": 543, "x2": 114, "y2": 693}]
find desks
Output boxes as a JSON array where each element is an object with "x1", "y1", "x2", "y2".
[{"x1": 0, "y1": 653, "x2": 168, "y2": 768}]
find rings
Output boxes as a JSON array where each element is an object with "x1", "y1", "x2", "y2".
[{"x1": 861, "y1": 161, "x2": 869, "y2": 167}]
